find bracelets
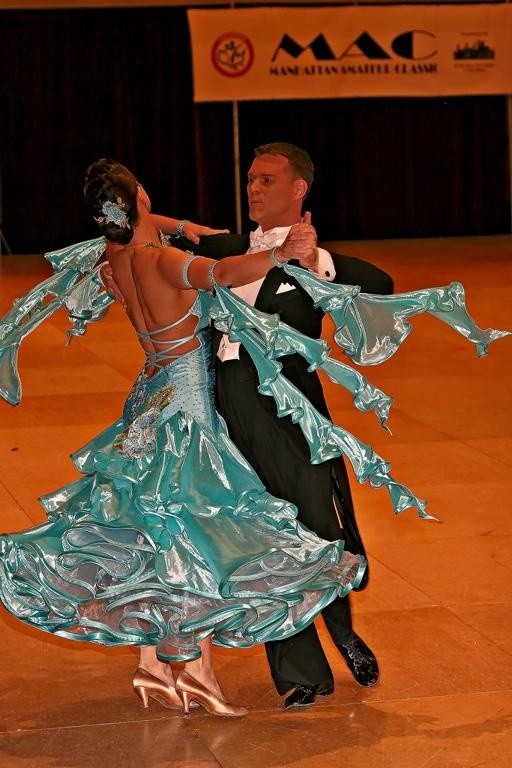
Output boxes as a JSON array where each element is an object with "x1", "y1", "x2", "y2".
[
  {"x1": 177, "y1": 220, "x2": 189, "y2": 237},
  {"x1": 270, "y1": 247, "x2": 287, "y2": 268}
]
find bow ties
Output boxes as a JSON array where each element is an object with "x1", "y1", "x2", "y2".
[{"x1": 249, "y1": 231, "x2": 276, "y2": 251}]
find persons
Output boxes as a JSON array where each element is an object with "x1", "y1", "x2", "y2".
[
  {"x1": 0, "y1": 158, "x2": 368, "y2": 720},
  {"x1": 98, "y1": 144, "x2": 394, "y2": 708}
]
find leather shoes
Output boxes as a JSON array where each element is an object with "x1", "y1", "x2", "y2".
[
  {"x1": 283, "y1": 683, "x2": 334, "y2": 709},
  {"x1": 337, "y1": 633, "x2": 380, "y2": 687}
]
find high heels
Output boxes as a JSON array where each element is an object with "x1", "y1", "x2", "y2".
[
  {"x1": 133, "y1": 666, "x2": 199, "y2": 710},
  {"x1": 175, "y1": 670, "x2": 248, "y2": 717}
]
